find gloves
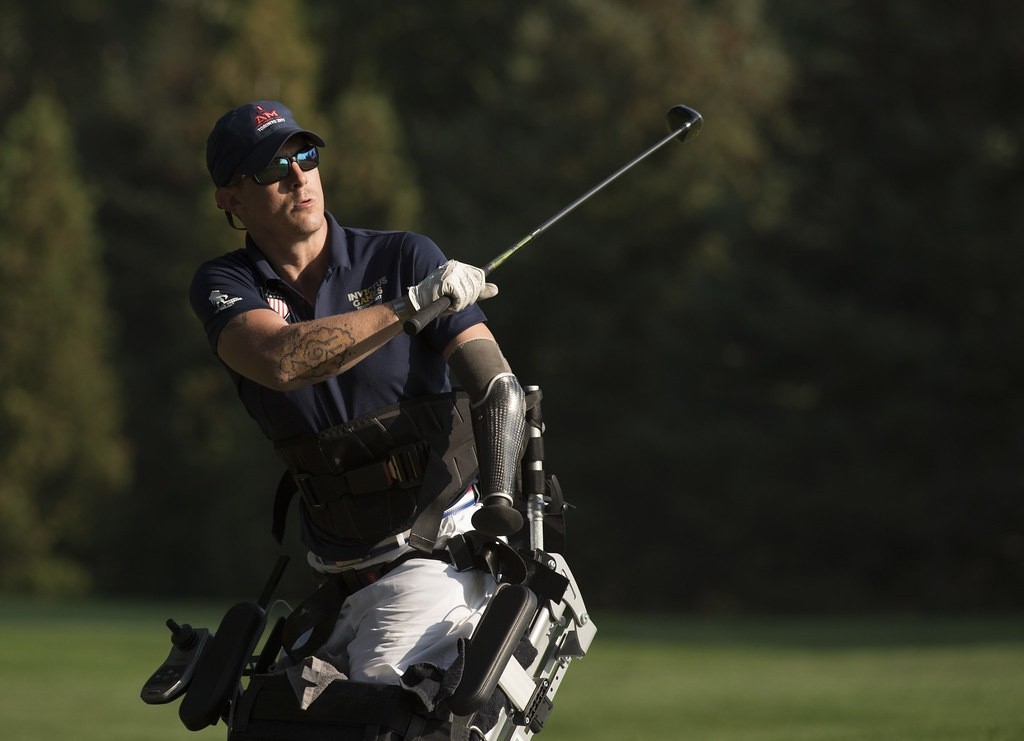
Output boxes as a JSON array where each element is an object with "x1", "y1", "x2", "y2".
[{"x1": 407, "y1": 259, "x2": 498, "y2": 315}]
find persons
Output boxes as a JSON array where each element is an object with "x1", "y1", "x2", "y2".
[{"x1": 189, "y1": 100, "x2": 527, "y2": 686}]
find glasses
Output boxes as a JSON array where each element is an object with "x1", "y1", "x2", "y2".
[{"x1": 252, "y1": 145, "x2": 320, "y2": 185}]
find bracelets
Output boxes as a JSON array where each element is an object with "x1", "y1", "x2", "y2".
[{"x1": 391, "y1": 296, "x2": 410, "y2": 323}]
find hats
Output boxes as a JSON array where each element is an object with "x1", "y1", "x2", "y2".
[{"x1": 206, "y1": 100, "x2": 326, "y2": 230}]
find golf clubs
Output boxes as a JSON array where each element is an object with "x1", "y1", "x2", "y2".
[{"x1": 404, "y1": 104, "x2": 704, "y2": 334}]
